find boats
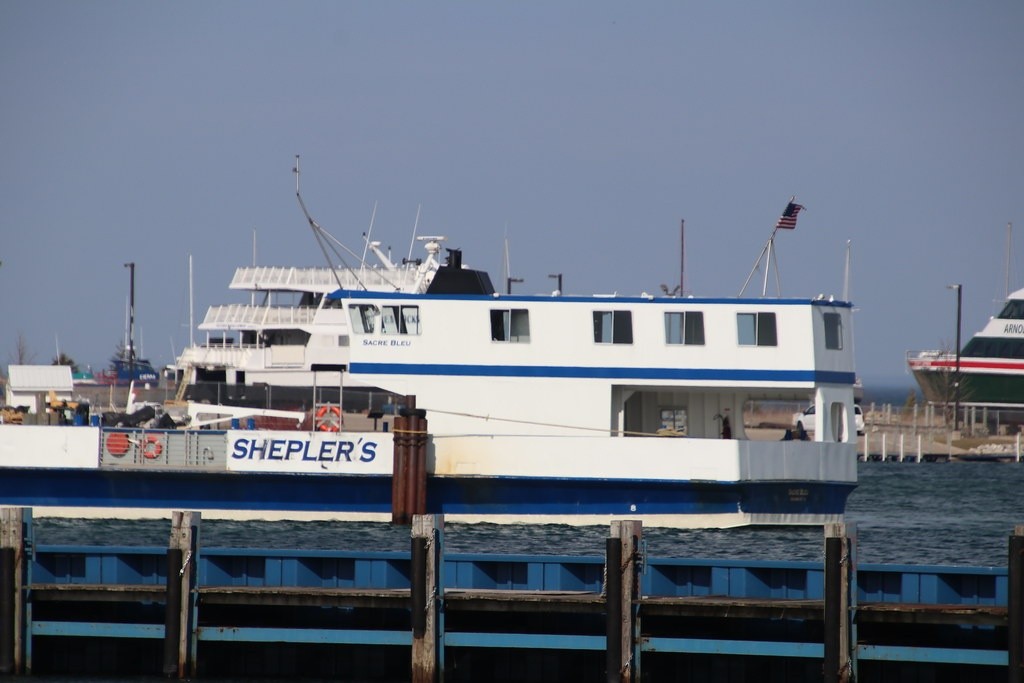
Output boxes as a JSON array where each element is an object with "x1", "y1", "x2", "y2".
[
  {"x1": 0, "y1": 151, "x2": 861, "y2": 529},
  {"x1": 909, "y1": 222, "x2": 1022, "y2": 424}
]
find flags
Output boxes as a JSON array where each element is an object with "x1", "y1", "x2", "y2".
[{"x1": 776, "y1": 203, "x2": 801, "y2": 229}]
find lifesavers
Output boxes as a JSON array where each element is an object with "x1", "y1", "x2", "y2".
[
  {"x1": 139, "y1": 436, "x2": 163, "y2": 460},
  {"x1": 315, "y1": 405, "x2": 343, "y2": 432}
]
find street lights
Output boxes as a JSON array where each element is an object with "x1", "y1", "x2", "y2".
[{"x1": 945, "y1": 284, "x2": 965, "y2": 431}]
